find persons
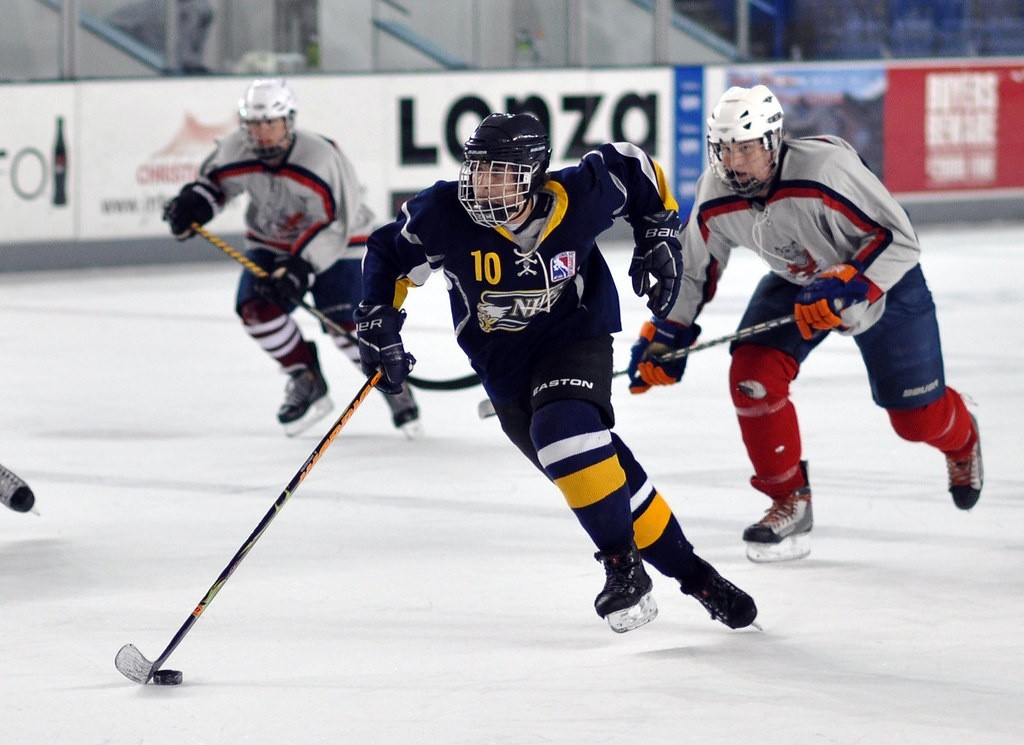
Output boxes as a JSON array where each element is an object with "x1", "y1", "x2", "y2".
[
  {"x1": 352, "y1": 112, "x2": 764, "y2": 630},
  {"x1": 629, "y1": 84, "x2": 985, "y2": 562},
  {"x1": 162, "y1": 77, "x2": 420, "y2": 441},
  {"x1": 0, "y1": 464, "x2": 41, "y2": 518}
]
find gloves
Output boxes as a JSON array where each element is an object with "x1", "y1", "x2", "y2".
[
  {"x1": 353, "y1": 300, "x2": 416, "y2": 394},
  {"x1": 795, "y1": 261, "x2": 872, "y2": 339},
  {"x1": 254, "y1": 258, "x2": 316, "y2": 314},
  {"x1": 163, "y1": 181, "x2": 219, "y2": 242},
  {"x1": 628, "y1": 210, "x2": 684, "y2": 319},
  {"x1": 628, "y1": 316, "x2": 701, "y2": 394}
]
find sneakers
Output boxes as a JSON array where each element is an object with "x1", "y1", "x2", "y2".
[
  {"x1": 594, "y1": 540, "x2": 658, "y2": 634},
  {"x1": 0, "y1": 465, "x2": 42, "y2": 518},
  {"x1": 278, "y1": 340, "x2": 334, "y2": 435},
  {"x1": 944, "y1": 411, "x2": 984, "y2": 510},
  {"x1": 742, "y1": 461, "x2": 814, "y2": 562},
  {"x1": 673, "y1": 552, "x2": 764, "y2": 633},
  {"x1": 385, "y1": 379, "x2": 419, "y2": 439}
]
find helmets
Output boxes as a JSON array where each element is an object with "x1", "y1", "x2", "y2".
[
  {"x1": 459, "y1": 112, "x2": 553, "y2": 228},
  {"x1": 706, "y1": 84, "x2": 784, "y2": 197},
  {"x1": 235, "y1": 81, "x2": 297, "y2": 161}
]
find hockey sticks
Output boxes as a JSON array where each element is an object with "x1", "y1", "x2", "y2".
[
  {"x1": 191, "y1": 222, "x2": 481, "y2": 390},
  {"x1": 478, "y1": 298, "x2": 843, "y2": 419},
  {"x1": 115, "y1": 366, "x2": 383, "y2": 685}
]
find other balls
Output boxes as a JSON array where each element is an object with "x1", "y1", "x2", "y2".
[{"x1": 152, "y1": 670, "x2": 183, "y2": 685}]
